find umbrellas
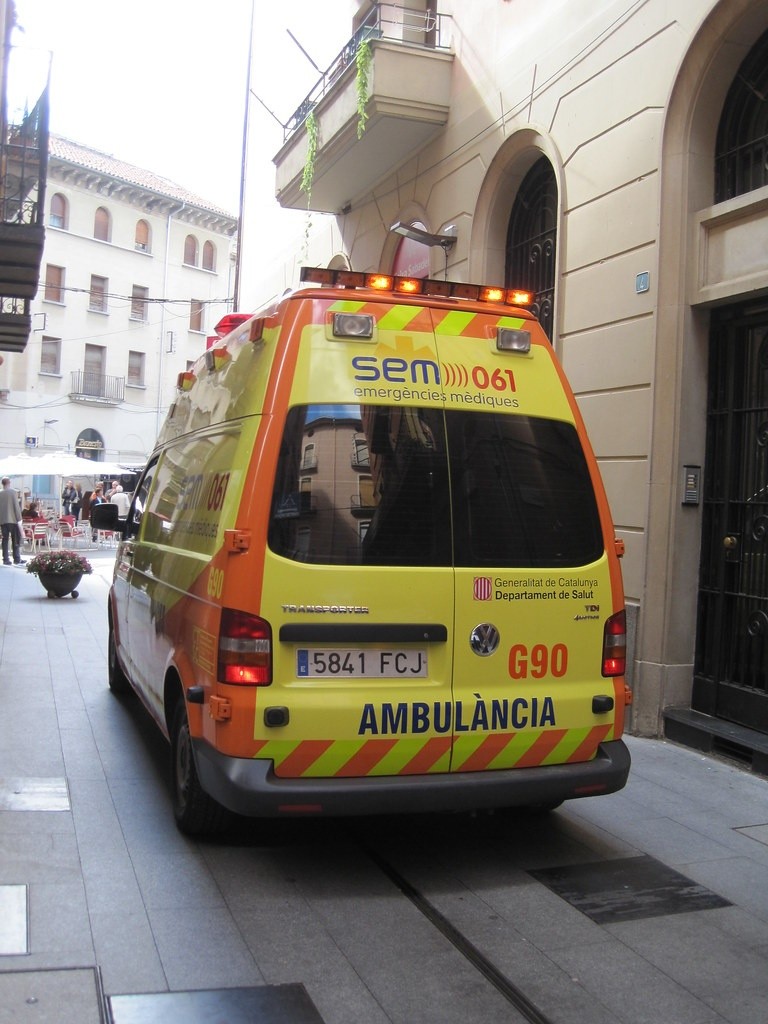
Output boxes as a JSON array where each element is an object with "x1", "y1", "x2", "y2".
[{"x1": 0, "y1": 451, "x2": 137, "y2": 518}]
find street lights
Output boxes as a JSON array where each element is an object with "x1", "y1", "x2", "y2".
[{"x1": 43, "y1": 417, "x2": 60, "y2": 446}]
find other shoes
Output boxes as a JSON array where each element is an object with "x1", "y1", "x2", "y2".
[
  {"x1": 3, "y1": 559, "x2": 12, "y2": 565},
  {"x1": 14, "y1": 559, "x2": 27, "y2": 564}
]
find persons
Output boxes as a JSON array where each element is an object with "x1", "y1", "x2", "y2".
[
  {"x1": 22, "y1": 502, "x2": 39, "y2": 519},
  {"x1": 0, "y1": 477, "x2": 26, "y2": 565},
  {"x1": 62, "y1": 481, "x2": 131, "y2": 543}
]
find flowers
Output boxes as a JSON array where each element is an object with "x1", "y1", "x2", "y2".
[{"x1": 26, "y1": 550, "x2": 94, "y2": 577}]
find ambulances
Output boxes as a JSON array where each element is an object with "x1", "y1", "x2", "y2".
[{"x1": 88, "y1": 266, "x2": 631, "y2": 842}]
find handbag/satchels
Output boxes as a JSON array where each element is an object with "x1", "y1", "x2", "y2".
[
  {"x1": 17, "y1": 520, "x2": 27, "y2": 539},
  {"x1": 70, "y1": 490, "x2": 78, "y2": 503}
]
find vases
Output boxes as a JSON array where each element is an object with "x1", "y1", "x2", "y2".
[
  {"x1": 39, "y1": 574, "x2": 82, "y2": 598},
  {"x1": 348, "y1": 26, "x2": 384, "y2": 61},
  {"x1": 297, "y1": 101, "x2": 317, "y2": 123}
]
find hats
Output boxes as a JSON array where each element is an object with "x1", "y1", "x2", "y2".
[{"x1": 116, "y1": 486, "x2": 123, "y2": 493}]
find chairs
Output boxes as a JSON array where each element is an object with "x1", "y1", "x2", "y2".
[{"x1": 22, "y1": 515, "x2": 116, "y2": 554}]
